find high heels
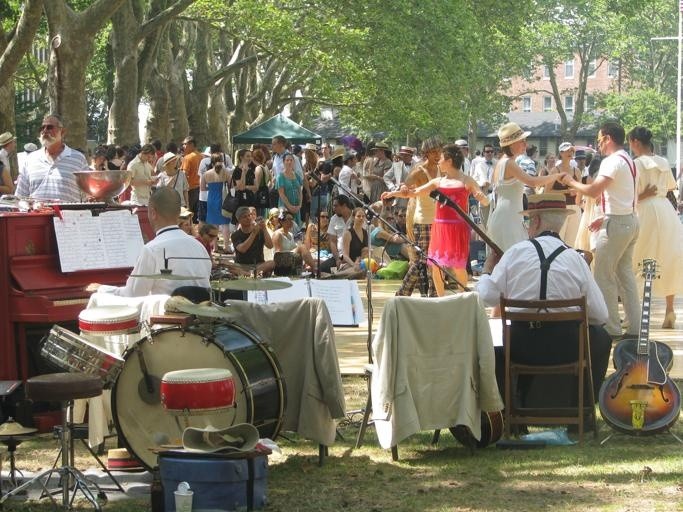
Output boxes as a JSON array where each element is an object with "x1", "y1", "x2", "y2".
[{"x1": 661, "y1": 311, "x2": 676, "y2": 329}]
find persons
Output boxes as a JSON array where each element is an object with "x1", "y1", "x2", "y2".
[
  {"x1": 475, "y1": 190, "x2": 613, "y2": 435},
  {"x1": 1, "y1": 112, "x2": 683, "y2": 341}
]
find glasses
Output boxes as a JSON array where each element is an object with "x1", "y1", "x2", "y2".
[
  {"x1": 484, "y1": 150, "x2": 495, "y2": 153},
  {"x1": 38, "y1": 124, "x2": 53, "y2": 130}
]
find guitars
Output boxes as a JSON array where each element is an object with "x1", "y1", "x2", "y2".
[
  {"x1": 450, "y1": 408, "x2": 504, "y2": 448},
  {"x1": 599, "y1": 257, "x2": 682, "y2": 436}
]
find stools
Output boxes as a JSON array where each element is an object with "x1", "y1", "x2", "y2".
[{"x1": 0, "y1": 367, "x2": 106, "y2": 512}]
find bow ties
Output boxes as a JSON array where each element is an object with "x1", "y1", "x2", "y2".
[{"x1": 486, "y1": 161, "x2": 492, "y2": 164}]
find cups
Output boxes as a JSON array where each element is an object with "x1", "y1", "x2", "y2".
[{"x1": 174, "y1": 490, "x2": 193, "y2": 512}]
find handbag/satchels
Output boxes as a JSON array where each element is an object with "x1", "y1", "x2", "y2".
[{"x1": 222, "y1": 185, "x2": 270, "y2": 213}]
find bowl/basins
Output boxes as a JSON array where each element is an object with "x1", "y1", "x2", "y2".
[
  {"x1": 72, "y1": 170, "x2": 133, "y2": 204},
  {"x1": 29, "y1": 408, "x2": 67, "y2": 434}
]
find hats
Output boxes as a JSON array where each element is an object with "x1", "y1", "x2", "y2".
[
  {"x1": 371, "y1": 141, "x2": 389, "y2": 150},
  {"x1": 455, "y1": 140, "x2": 468, "y2": 148},
  {"x1": 0, "y1": 133, "x2": 17, "y2": 146},
  {"x1": 497, "y1": 123, "x2": 532, "y2": 147},
  {"x1": 559, "y1": 141, "x2": 576, "y2": 153},
  {"x1": 421, "y1": 136, "x2": 443, "y2": 152},
  {"x1": 397, "y1": 146, "x2": 415, "y2": 155},
  {"x1": 519, "y1": 193, "x2": 575, "y2": 215},
  {"x1": 161, "y1": 152, "x2": 178, "y2": 168}
]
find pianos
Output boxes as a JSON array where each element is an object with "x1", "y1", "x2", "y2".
[{"x1": 0, "y1": 206, "x2": 157, "y2": 430}]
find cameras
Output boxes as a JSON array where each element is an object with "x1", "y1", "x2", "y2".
[{"x1": 256, "y1": 217, "x2": 261, "y2": 224}]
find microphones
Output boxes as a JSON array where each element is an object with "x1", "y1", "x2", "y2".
[{"x1": 329, "y1": 175, "x2": 342, "y2": 189}]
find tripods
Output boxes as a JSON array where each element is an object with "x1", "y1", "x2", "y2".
[{"x1": 1, "y1": 418, "x2": 100, "y2": 512}]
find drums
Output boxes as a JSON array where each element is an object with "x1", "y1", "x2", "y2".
[
  {"x1": 79, "y1": 304, "x2": 141, "y2": 336},
  {"x1": 112, "y1": 316, "x2": 287, "y2": 472},
  {"x1": 161, "y1": 368, "x2": 236, "y2": 411},
  {"x1": 39, "y1": 325, "x2": 124, "y2": 389}
]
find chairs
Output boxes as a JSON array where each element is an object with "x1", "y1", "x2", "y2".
[
  {"x1": 66, "y1": 287, "x2": 177, "y2": 456},
  {"x1": 353, "y1": 291, "x2": 494, "y2": 461},
  {"x1": 497, "y1": 289, "x2": 601, "y2": 449},
  {"x1": 230, "y1": 298, "x2": 350, "y2": 468}
]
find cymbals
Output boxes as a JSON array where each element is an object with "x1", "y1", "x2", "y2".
[
  {"x1": 126, "y1": 272, "x2": 204, "y2": 281},
  {"x1": 178, "y1": 301, "x2": 242, "y2": 319},
  {"x1": 212, "y1": 279, "x2": 293, "y2": 291}
]
